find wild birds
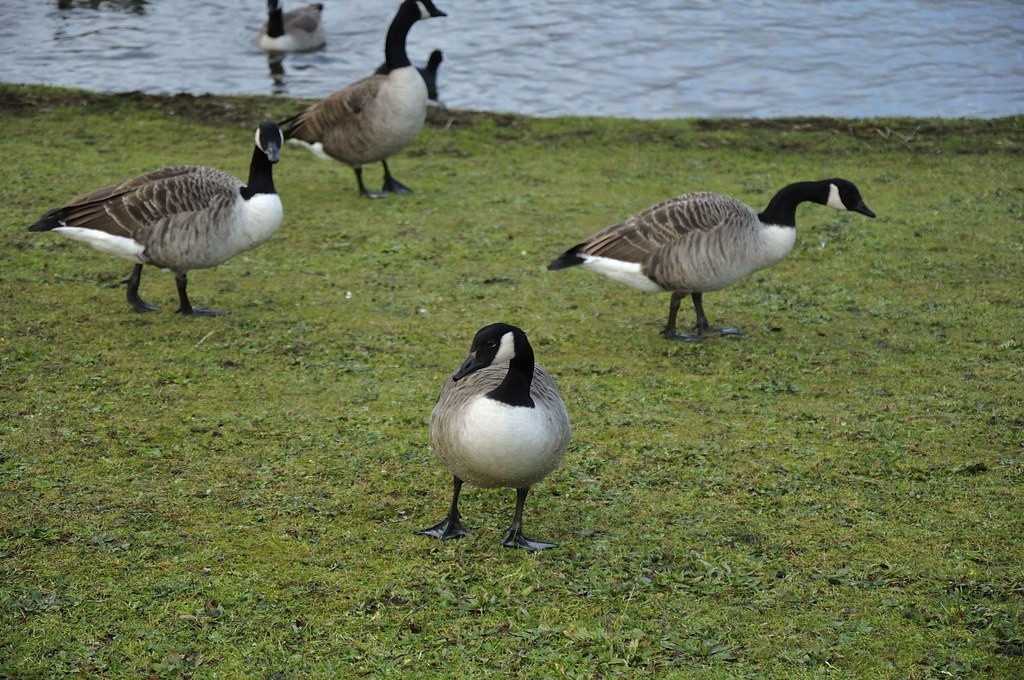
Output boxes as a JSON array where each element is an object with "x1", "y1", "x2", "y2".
[
  {"x1": 547, "y1": 176, "x2": 877, "y2": 342},
  {"x1": 256, "y1": 0, "x2": 327, "y2": 53},
  {"x1": 27, "y1": 123, "x2": 286, "y2": 317},
  {"x1": 275, "y1": 0, "x2": 449, "y2": 196},
  {"x1": 411, "y1": 323, "x2": 572, "y2": 554}
]
